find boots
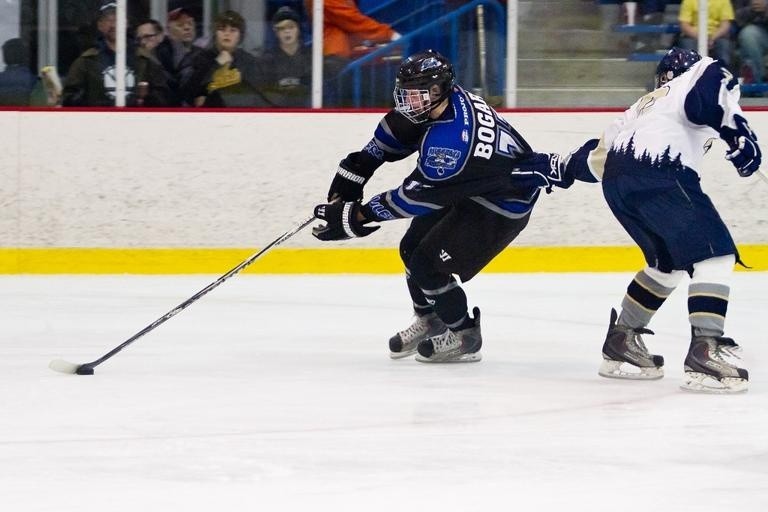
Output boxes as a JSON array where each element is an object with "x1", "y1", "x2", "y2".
[
  {"x1": 417, "y1": 307, "x2": 482, "y2": 362},
  {"x1": 389, "y1": 307, "x2": 446, "y2": 358},
  {"x1": 685, "y1": 327, "x2": 748, "y2": 378},
  {"x1": 603, "y1": 308, "x2": 664, "y2": 367}
]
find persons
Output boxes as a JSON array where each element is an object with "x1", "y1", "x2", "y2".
[
  {"x1": 445, "y1": 2, "x2": 503, "y2": 108},
  {"x1": 632, "y1": 1, "x2": 767, "y2": 84},
  {"x1": 1, "y1": 1, "x2": 403, "y2": 109},
  {"x1": 509, "y1": 45, "x2": 762, "y2": 393},
  {"x1": 311, "y1": 50, "x2": 539, "y2": 364}
]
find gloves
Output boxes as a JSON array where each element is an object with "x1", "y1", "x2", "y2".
[
  {"x1": 328, "y1": 153, "x2": 375, "y2": 203},
  {"x1": 512, "y1": 149, "x2": 573, "y2": 193},
  {"x1": 725, "y1": 116, "x2": 761, "y2": 176},
  {"x1": 313, "y1": 202, "x2": 379, "y2": 241}
]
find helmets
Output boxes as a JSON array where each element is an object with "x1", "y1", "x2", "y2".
[
  {"x1": 393, "y1": 51, "x2": 455, "y2": 124},
  {"x1": 654, "y1": 47, "x2": 702, "y2": 89}
]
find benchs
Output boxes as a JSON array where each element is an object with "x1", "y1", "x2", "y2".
[{"x1": 611, "y1": 23, "x2": 768, "y2": 92}]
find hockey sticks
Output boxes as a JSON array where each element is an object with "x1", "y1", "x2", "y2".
[{"x1": 49, "y1": 196, "x2": 342, "y2": 375}]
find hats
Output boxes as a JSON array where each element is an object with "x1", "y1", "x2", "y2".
[
  {"x1": 169, "y1": 7, "x2": 192, "y2": 19},
  {"x1": 271, "y1": 7, "x2": 300, "y2": 25}
]
